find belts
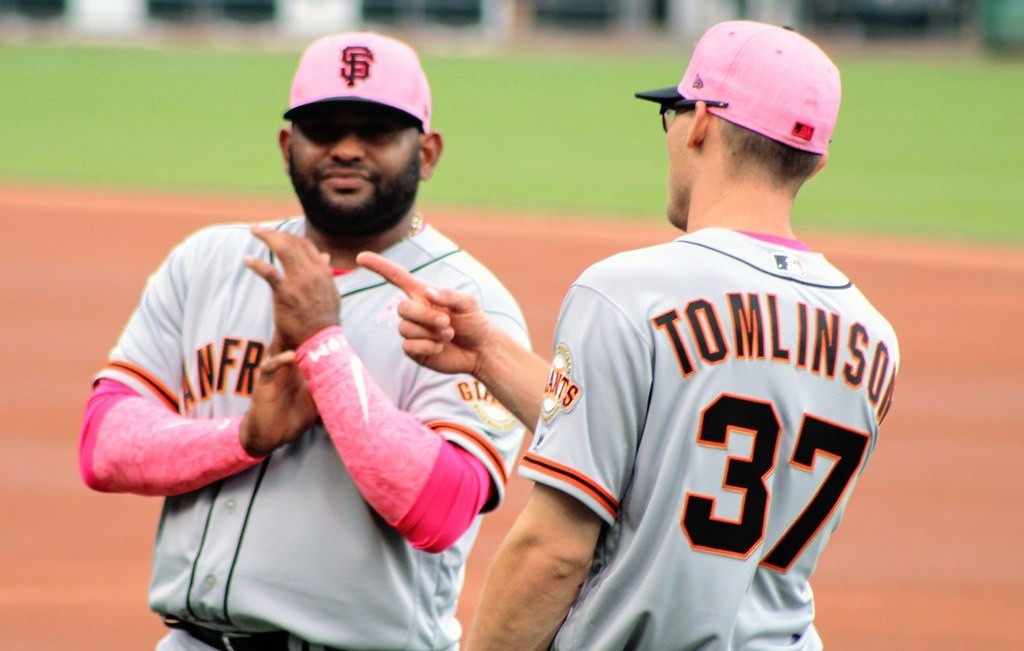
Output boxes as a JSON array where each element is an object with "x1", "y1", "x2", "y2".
[{"x1": 164, "y1": 614, "x2": 345, "y2": 651}]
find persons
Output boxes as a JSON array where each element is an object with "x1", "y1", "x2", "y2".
[
  {"x1": 79, "y1": 32, "x2": 532, "y2": 651},
  {"x1": 356, "y1": 20, "x2": 900, "y2": 651}
]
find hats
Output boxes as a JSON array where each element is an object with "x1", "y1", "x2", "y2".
[
  {"x1": 283, "y1": 32, "x2": 431, "y2": 134},
  {"x1": 635, "y1": 20, "x2": 842, "y2": 156}
]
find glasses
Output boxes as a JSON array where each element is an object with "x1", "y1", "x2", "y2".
[{"x1": 660, "y1": 100, "x2": 729, "y2": 133}]
